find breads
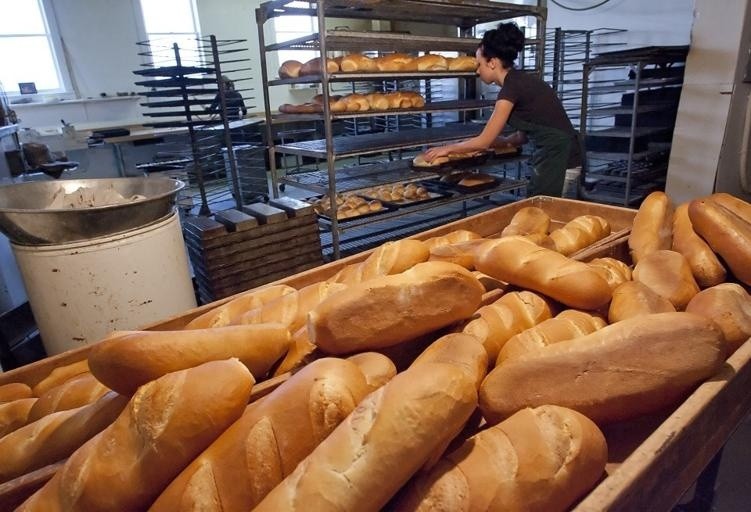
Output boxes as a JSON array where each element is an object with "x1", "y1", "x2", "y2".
[
  {"x1": 412, "y1": 144, "x2": 517, "y2": 167},
  {"x1": 278, "y1": 54, "x2": 480, "y2": 79},
  {"x1": 1, "y1": 191, "x2": 751, "y2": 512},
  {"x1": 279, "y1": 91, "x2": 424, "y2": 113}
]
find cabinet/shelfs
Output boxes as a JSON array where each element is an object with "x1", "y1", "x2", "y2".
[
  {"x1": 580, "y1": 49, "x2": 693, "y2": 205},
  {"x1": 256, "y1": 0, "x2": 548, "y2": 265},
  {"x1": 521, "y1": 27, "x2": 628, "y2": 160}
]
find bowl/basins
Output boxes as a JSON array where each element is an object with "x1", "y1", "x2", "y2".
[{"x1": 1, "y1": 177, "x2": 184, "y2": 248}]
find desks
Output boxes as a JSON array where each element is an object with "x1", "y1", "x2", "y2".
[{"x1": 1, "y1": 194, "x2": 751, "y2": 512}]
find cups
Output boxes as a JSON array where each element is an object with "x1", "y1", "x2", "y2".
[{"x1": 61, "y1": 126, "x2": 74, "y2": 138}]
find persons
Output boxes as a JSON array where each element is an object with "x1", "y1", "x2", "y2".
[
  {"x1": 427, "y1": 20, "x2": 587, "y2": 200},
  {"x1": 204, "y1": 76, "x2": 249, "y2": 146}
]
